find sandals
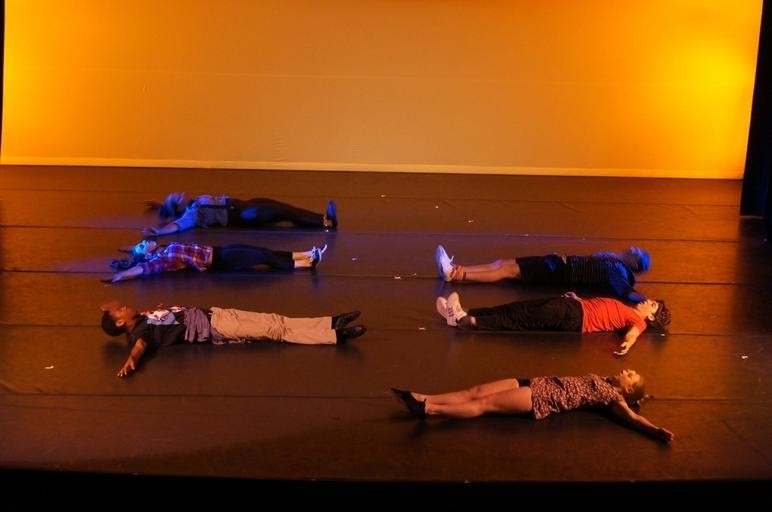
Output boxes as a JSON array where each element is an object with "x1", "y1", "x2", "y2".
[{"x1": 390, "y1": 387, "x2": 426, "y2": 418}]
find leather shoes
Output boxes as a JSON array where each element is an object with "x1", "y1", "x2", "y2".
[{"x1": 332, "y1": 311, "x2": 364, "y2": 340}]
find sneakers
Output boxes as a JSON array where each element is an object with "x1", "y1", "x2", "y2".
[
  {"x1": 321, "y1": 201, "x2": 337, "y2": 229},
  {"x1": 435, "y1": 292, "x2": 467, "y2": 327},
  {"x1": 435, "y1": 244, "x2": 457, "y2": 282},
  {"x1": 310, "y1": 243, "x2": 328, "y2": 269}
]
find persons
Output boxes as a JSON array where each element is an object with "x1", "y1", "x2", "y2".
[
  {"x1": 390, "y1": 367, "x2": 674, "y2": 445},
  {"x1": 142, "y1": 190, "x2": 337, "y2": 238},
  {"x1": 434, "y1": 244, "x2": 649, "y2": 302},
  {"x1": 99, "y1": 299, "x2": 366, "y2": 378},
  {"x1": 434, "y1": 291, "x2": 671, "y2": 357},
  {"x1": 100, "y1": 240, "x2": 327, "y2": 285}
]
